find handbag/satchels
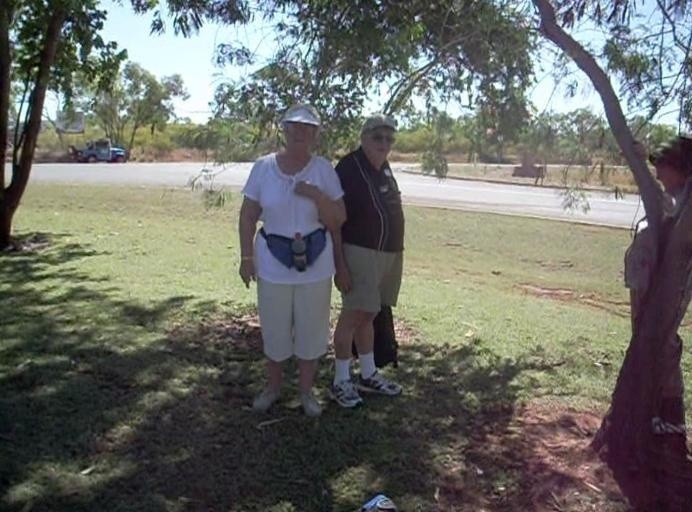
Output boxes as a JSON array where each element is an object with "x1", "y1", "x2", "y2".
[
  {"x1": 353, "y1": 305, "x2": 398, "y2": 368},
  {"x1": 261, "y1": 227, "x2": 326, "y2": 269}
]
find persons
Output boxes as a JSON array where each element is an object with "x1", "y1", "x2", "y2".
[
  {"x1": 239, "y1": 104, "x2": 347, "y2": 418},
  {"x1": 625, "y1": 135, "x2": 692, "y2": 437},
  {"x1": 330, "y1": 112, "x2": 405, "y2": 410}
]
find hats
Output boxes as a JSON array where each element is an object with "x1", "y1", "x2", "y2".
[
  {"x1": 282, "y1": 108, "x2": 320, "y2": 126},
  {"x1": 649, "y1": 135, "x2": 690, "y2": 171},
  {"x1": 364, "y1": 113, "x2": 395, "y2": 131}
]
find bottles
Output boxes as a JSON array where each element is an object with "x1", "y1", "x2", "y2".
[{"x1": 291, "y1": 229, "x2": 310, "y2": 275}]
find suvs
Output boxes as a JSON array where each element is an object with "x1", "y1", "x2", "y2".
[{"x1": 71, "y1": 138, "x2": 127, "y2": 164}]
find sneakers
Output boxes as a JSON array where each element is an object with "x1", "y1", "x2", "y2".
[
  {"x1": 301, "y1": 392, "x2": 323, "y2": 417},
  {"x1": 358, "y1": 370, "x2": 402, "y2": 396},
  {"x1": 253, "y1": 390, "x2": 280, "y2": 409},
  {"x1": 654, "y1": 421, "x2": 687, "y2": 435},
  {"x1": 327, "y1": 379, "x2": 363, "y2": 408}
]
